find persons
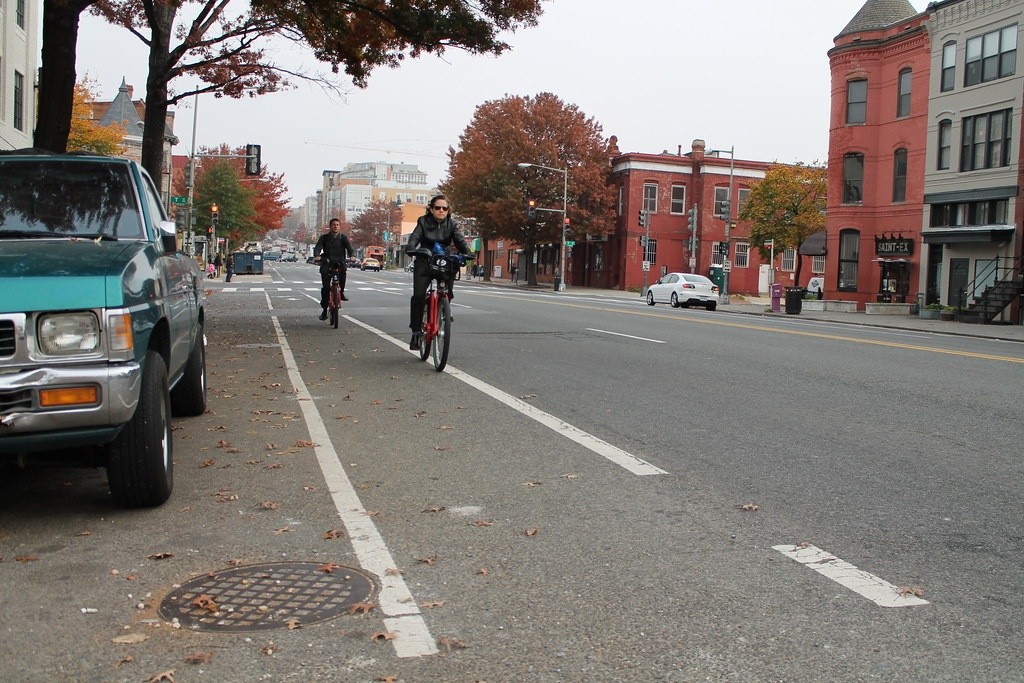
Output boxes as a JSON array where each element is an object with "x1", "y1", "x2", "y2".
[
  {"x1": 314, "y1": 219, "x2": 356, "y2": 321},
  {"x1": 405, "y1": 194, "x2": 475, "y2": 351},
  {"x1": 208, "y1": 252, "x2": 221, "y2": 279},
  {"x1": 512, "y1": 263, "x2": 516, "y2": 282},
  {"x1": 226, "y1": 254, "x2": 234, "y2": 282},
  {"x1": 473, "y1": 264, "x2": 477, "y2": 278}
]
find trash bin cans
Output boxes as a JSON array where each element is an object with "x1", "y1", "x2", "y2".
[
  {"x1": 784, "y1": 286, "x2": 802, "y2": 314},
  {"x1": 233, "y1": 251, "x2": 263, "y2": 275},
  {"x1": 554, "y1": 276, "x2": 561, "y2": 291}
]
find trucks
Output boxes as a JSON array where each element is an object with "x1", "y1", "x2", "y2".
[{"x1": 365, "y1": 246, "x2": 384, "y2": 269}]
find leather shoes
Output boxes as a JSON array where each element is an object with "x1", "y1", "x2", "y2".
[
  {"x1": 443, "y1": 306, "x2": 454, "y2": 322},
  {"x1": 340, "y1": 292, "x2": 349, "y2": 301},
  {"x1": 319, "y1": 310, "x2": 327, "y2": 320},
  {"x1": 410, "y1": 333, "x2": 421, "y2": 350}
]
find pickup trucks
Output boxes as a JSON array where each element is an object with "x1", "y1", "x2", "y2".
[{"x1": 0, "y1": 146, "x2": 208, "y2": 510}]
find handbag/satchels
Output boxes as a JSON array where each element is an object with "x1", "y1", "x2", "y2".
[{"x1": 320, "y1": 253, "x2": 328, "y2": 259}]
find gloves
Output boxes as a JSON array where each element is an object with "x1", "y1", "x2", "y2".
[
  {"x1": 465, "y1": 252, "x2": 476, "y2": 260},
  {"x1": 406, "y1": 245, "x2": 416, "y2": 256}
]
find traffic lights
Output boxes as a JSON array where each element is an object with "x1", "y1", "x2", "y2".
[
  {"x1": 211, "y1": 205, "x2": 219, "y2": 224},
  {"x1": 528, "y1": 199, "x2": 536, "y2": 219},
  {"x1": 719, "y1": 200, "x2": 727, "y2": 221},
  {"x1": 687, "y1": 209, "x2": 693, "y2": 230},
  {"x1": 565, "y1": 217, "x2": 571, "y2": 236},
  {"x1": 637, "y1": 209, "x2": 645, "y2": 227},
  {"x1": 185, "y1": 163, "x2": 190, "y2": 187},
  {"x1": 719, "y1": 241, "x2": 728, "y2": 255}
]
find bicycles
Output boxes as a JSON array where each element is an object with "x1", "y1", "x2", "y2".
[
  {"x1": 405, "y1": 248, "x2": 475, "y2": 373},
  {"x1": 310, "y1": 259, "x2": 353, "y2": 329}
]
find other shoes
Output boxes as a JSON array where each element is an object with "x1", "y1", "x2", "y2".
[{"x1": 226, "y1": 281, "x2": 231, "y2": 282}]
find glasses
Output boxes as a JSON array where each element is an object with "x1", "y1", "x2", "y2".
[{"x1": 432, "y1": 205, "x2": 448, "y2": 211}]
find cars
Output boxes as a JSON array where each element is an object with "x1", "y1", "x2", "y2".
[
  {"x1": 361, "y1": 258, "x2": 380, "y2": 272},
  {"x1": 645, "y1": 272, "x2": 719, "y2": 311},
  {"x1": 264, "y1": 238, "x2": 361, "y2": 269}
]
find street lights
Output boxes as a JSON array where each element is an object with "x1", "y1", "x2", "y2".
[
  {"x1": 704, "y1": 144, "x2": 734, "y2": 304},
  {"x1": 517, "y1": 163, "x2": 568, "y2": 291}
]
